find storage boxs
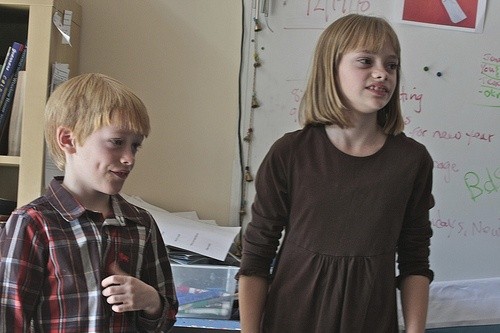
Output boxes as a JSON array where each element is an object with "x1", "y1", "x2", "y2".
[{"x1": 170, "y1": 252, "x2": 242, "y2": 320}]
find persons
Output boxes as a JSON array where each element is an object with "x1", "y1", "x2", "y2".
[
  {"x1": 233, "y1": 14, "x2": 434, "y2": 333},
  {"x1": 0, "y1": 74, "x2": 179, "y2": 333}
]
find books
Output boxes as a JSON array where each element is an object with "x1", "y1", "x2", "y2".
[{"x1": 0, "y1": 42, "x2": 27, "y2": 156}]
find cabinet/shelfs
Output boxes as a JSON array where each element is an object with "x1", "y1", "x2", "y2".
[{"x1": 0, "y1": 0, "x2": 83, "y2": 230}]
